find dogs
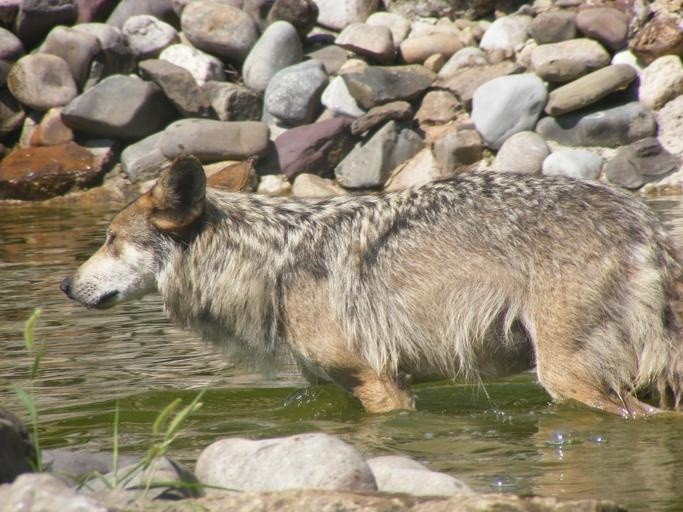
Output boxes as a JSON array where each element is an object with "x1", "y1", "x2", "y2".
[{"x1": 56, "y1": 152, "x2": 683, "y2": 422}]
